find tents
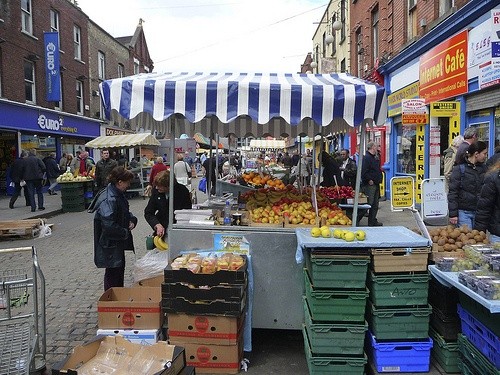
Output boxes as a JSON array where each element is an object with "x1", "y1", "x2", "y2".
[
  {"x1": 85, "y1": 132, "x2": 161, "y2": 148},
  {"x1": 99, "y1": 72, "x2": 388, "y2": 230}
]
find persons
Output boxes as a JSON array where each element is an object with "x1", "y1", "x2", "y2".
[
  {"x1": 486, "y1": 146, "x2": 500, "y2": 167},
  {"x1": 402, "y1": 149, "x2": 415, "y2": 174},
  {"x1": 276, "y1": 144, "x2": 360, "y2": 220},
  {"x1": 93, "y1": 166, "x2": 138, "y2": 291},
  {"x1": 448, "y1": 141, "x2": 488, "y2": 229},
  {"x1": 444, "y1": 135, "x2": 464, "y2": 190},
  {"x1": 59, "y1": 153, "x2": 68, "y2": 174},
  {"x1": 70, "y1": 151, "x2": 82, "y2": 176},
  {"x1": 81, "y1": 151, "x2": 95, "y2": 208},
  {"x1": 454, "y1": 127, "x2": 478, "y2": 165},
  {"x1": 79, "y1": 152, "x2": 86, "y2": 176},
  {"x1": 94, "y1": 149, "x2": 117, "y2": 203},
  {"x1": 46, "y1": 152, "x2": 61, "y2": 195},
  {"x1": 473, "y1": 164, "x2": 500, "y2": 237},
  {"x1": 66, "y1": 153, "x2": 74, "y2": 172},
  {"x1": 20, "y1": 149, "x2": 46, "y2": 212},
  {"x1": 111, "y1": 149, "x2": 242, "y2": 195},
  {"x1": 9, "y1": 152, "x2": 31, "y2": 209},
  {"x1": 356, "y1": 141, "x2": 383, "y2": 227},
  {"x1": 144, "y1": 171, "x2": 192, "y2": 249}
]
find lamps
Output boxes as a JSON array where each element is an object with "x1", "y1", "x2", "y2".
[{"x1": 306, "y1": 21, "x2": 342, "y2": 74}]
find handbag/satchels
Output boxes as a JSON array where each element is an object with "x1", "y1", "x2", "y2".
[
  {"x1": 187, "y1": 172, "x2": 192, "y2": 178},
  {"x1": 144, "y1": 185, "x2": 152, "y2": 196},
  {"x1": 198, "y1": 177, "x2": 207, "y2": 193}
]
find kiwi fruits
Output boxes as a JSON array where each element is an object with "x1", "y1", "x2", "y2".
[{"x1": 428, "y1": 223, "x2": 490, "y2": 252}]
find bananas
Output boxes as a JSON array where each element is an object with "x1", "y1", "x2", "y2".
[
  {"x1": 154, "y1": 236, "x2": 168, "y2": 251},
  {"x1": 245, "y1": 190, "x2": 324, "y2": 209}
]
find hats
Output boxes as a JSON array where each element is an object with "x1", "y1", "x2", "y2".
[{"x1": 29, "y1": 149, "x2": 36, "y2": 155}]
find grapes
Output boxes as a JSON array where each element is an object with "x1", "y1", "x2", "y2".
[{"x1": 435, "y1": 244, "x2": 500, "y2": 301}]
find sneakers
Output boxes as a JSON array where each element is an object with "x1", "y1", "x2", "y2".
[{"x1": 368, "y1": 221, "x2": 383, "y2": 226}]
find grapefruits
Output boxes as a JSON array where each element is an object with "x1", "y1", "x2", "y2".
[{"x1": 242, "y1": 172, "x2": 285, "y2": 189}]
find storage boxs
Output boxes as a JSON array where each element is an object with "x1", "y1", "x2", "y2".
[
  {"x1": 50, "y1": 254, "x2": 248, "y2": 375},
  {"x1": 61, "y1": 181, "x2": 94, "y2": 213},
  {"x1": 302, "y1": 246, "x2": 500, "y2": 375}
]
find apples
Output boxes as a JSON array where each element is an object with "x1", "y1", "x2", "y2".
[
  {"x1": 249, "y1": 197, "x2": 353, "y2": 225},
  {"x1": 239, "y1": 183, "x2": 298, "y2": 200},
  {"x1": 311, "y1": 226, "x2": 366, "y2": 241}
]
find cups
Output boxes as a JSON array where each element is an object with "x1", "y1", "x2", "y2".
[{"x1": 233, "y1": 214, "x2": 242, "y2": 226}]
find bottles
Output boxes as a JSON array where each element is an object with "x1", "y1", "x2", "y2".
[{"x1": 225, "y1": 201, "x2": 231, "y2": 226}]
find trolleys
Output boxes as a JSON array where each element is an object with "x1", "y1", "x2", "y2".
[{"x1": 0, "y1": 246, "x2": 46, "y2": 375}]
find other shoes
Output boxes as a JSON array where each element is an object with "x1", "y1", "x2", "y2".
[
  {"x1": 38, "y1": 207, "x2": 45, "y2": 210},
  {"x1": 26, "y1": 202, "x2": 31, "y2": 206},
  {"x1": 52, "y1": 193, "x2": 57, "y2": 195},
  {"x1": 9, "y1": 203, "x2": 14, "y2": 209},
  {"x1": 47, "y1": 189, "x2": 51, "y2": 195}
]
